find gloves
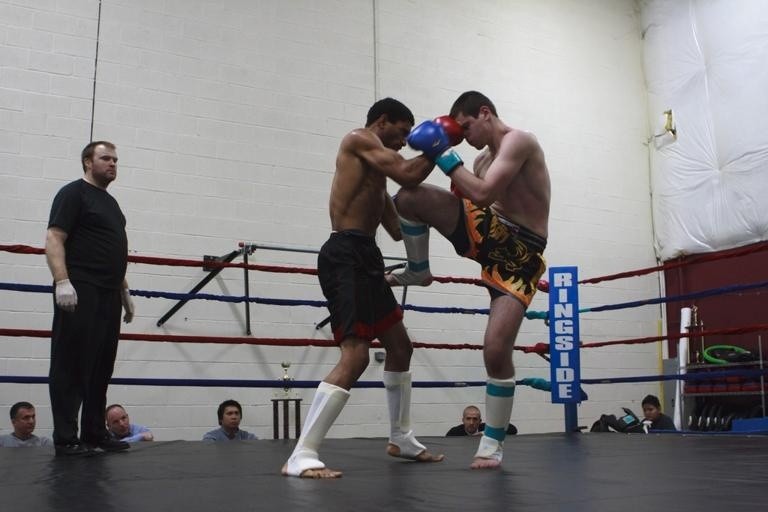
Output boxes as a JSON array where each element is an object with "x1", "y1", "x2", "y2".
[
  {"x1": 432, "y1": 116, "x2": 465, "y2": 146},
  {"x1": 405, "y1": 121, "x2": 464, "y2": 175},
  {"x1": 55, "y1": 279, "x2": 77, "y2": 313},
  {"x1": 121, "y1": 288, "x2": 134, "y2": 324}
]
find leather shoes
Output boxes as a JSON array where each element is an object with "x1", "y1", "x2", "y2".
[
  {"x1": 81, "y1": 431, "x2": 131, "y2": 451},
  {"x1": 54, "y1": 438, "x2": 97, "y2": 458}
]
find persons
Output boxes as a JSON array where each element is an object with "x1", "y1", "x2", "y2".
[
  {"x1": 637, "y1": 394, "x2": 679, "y2": 433},
  {"x1": 1, "y1": 401, "x2": 56, "y2": 447},
  {"x1": 444, "y1": 405, "x2": 518, "y2": 438},
  {"x1": 281, "y1": 97, "x2": 465, "y2": 480},
  {"x1": 204, "y1": 398, "x2": 262, "y2": 440},
  {"x1": 383, "y1": 91, "x2": 552, "y2": 471},
  {"x1": 103, "y1": 403, "x2": 156, "y2": 444},
  {"x1": 43, "y1": 140, "x2": 136, "y2": 457}
]
